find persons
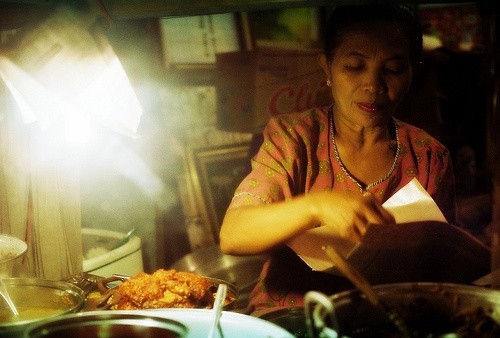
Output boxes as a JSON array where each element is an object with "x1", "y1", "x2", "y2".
[{"x1": 218, "y1": 1, "x2": 452, "y2": 315}]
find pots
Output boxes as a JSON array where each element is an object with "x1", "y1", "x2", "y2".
[{"x1": 303, "y1": 280, "x2": 499, "y2": 338}]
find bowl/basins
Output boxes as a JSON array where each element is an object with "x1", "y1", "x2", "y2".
[
  {"x1": 20, "y1": 310, "x2": 191, "y2": 338},
  {"x1": 96, "y1": 270, "x2": 241, "y2": 314},
  {"x1": 0, "y1": 232, "x2": 29, "y2": 276},
  {"x1": 1, "y1": 274, "x2": 85, "y2": 338}
]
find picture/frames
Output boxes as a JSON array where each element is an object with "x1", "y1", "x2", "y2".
[
  {"x1": 148, "y1": 11, "x2": 255, "y2": 88},
  {"x1": 184, "y1": 136, "x2": 254, "y2": 245}
]
